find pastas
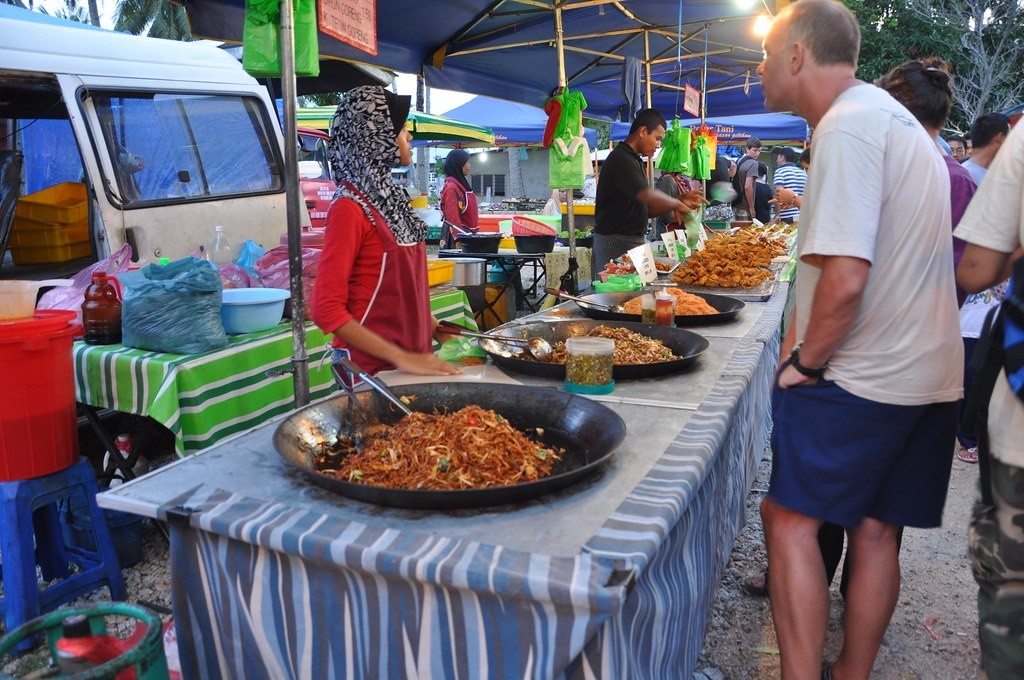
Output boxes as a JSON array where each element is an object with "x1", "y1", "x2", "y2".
[{"x1": 321, "y1": 403, "x2": 558, "y2": 490}]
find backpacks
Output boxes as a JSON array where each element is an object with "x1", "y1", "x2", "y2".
[{"x1": 730, "y1": 156, "x2": 753, "y2": 207}]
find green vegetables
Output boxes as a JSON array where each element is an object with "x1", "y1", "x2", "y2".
[{"x1": 558, "y1": 228, "x2": 592, "y2": 238}]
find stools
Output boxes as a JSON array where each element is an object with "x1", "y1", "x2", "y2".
[{"x1": 0, "y1": 457, "x2": 128, "y2": 653}]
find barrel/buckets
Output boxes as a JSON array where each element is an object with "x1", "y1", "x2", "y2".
[
  {"x1": 475, "y1": 282, "x2": 517, "y2": 330},
  {"x1": 0, "y1": 309, "x2": 80, "y2": 482}
]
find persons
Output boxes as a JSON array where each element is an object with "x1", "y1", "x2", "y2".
[
  {"x1": 590, "y1": 109, "x2": 811, "y2": 281},
  {"x1": 439, "y1": 149, "x2": 480, "y2": 250},
  {"x1": 544, "y1": 188, "x2": 569, "y2": 246},
  {"x1": 309, "y1": 86, "x2": 487, "y2": 389},
  {"x1": 755, "y1": 0, "x2": 1024, "y2": 680}
]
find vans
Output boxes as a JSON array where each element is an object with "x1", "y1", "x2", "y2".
[{"x1": 0, "y1": 2, "x2": 315, "y2": 298}]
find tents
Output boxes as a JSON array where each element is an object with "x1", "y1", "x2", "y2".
[
  {"x1": 167, "y1": 0, "x2": 793, "y2": 413},
  {"x1": 297, "y1": 106, "x2": 496, "y2": 200},
  {"x1": 411, "y1": 95, "x2": 807, "y2": 195}
]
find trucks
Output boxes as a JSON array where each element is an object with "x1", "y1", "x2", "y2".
[{"x1": 295, "y1": 127, "x2": 340, "y2": 234}]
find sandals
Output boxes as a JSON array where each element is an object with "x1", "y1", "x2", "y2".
[{"x1": 743, "y1": 566, "x2": 771, "y2": 592}]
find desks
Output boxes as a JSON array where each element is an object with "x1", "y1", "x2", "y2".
[{"x1": 71, "y1": 197, "x2": 800, "y2": 680}]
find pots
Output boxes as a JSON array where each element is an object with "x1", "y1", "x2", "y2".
[
  {"x1": 454, "y1": 231, "x2": 503, "y2": 252},
  {"x1": 272, "y1": 382, "x2": 625, "y2": 509},
  {"x1": 477, "y1": 321, "x2": 710, "y2": 380},
  {"x1": 514, "y1": 234, "x2": 555, "y2": 254},
  {"x1": 575, "y1": 290, "x2": 745, "y2": 327},
  {"x1": 438, "y1": 257, "x2": 489, "y2": 286}
]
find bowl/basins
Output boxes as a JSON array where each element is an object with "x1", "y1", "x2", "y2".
[
  {"x1": 222, "y1": 288, "x2": 292, "y2": 334},
  {"x1": 563, "y1": 235, "x2": 593, "y2": 247}
]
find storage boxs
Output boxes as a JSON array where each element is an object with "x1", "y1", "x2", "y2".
[
  {"x1": 8, "y1": 182, "x2": 92, "y2": 279},
  {"x1": 478, "y1": 214, "x2": 514, "y2": 232},
  {"x1": 427, "y1": 261, "x2": 456, "y2": 287},
  {"x1": 500, "y1": 238, "x2": 516, "y2": 249}
]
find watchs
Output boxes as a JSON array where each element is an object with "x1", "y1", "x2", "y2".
[{"x1": 789, "y1": 342, "x2": 829, "y2": 378}]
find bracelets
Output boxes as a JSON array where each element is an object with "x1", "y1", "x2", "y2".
[{"x1": 792, "y1": 193, "x2": 798, "y2": 205}]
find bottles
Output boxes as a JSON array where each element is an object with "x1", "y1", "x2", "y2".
[
  {"x1": 210, "y1": 225, "x2": 238, "y2": 266},
  {"x1": 103, "y1": 434, "x2": 149, "y2": 489},
  {"x1": 641, "y1": 288, "x2": 677, "y2": 328},
  {"x1": 81, "y1": 271, "x2": 122, "y2": 345}
]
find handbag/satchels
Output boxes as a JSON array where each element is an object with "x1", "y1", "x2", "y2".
[
  {"x1": 35, "y1": 242, "x2": 131, "y2": 328},
  {"x1": 683, "y1": 204, "x2": 703, "y2": 247}
]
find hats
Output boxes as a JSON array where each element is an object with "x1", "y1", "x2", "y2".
[{"x1": 383, "y1": 88, "x2": 411, "y2": 138}]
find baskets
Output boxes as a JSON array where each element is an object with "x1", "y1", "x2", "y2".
[{"x1": 512, "y1": 216, "x2": 556, "y2": 236}]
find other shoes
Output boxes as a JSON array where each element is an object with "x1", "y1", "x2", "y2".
[
  {"x1": 822, "y1": 660, "x2": 834, "y2": 680},
  {"x1": 957, "y1": 448, "x2": 978, "y2": 463}
]
